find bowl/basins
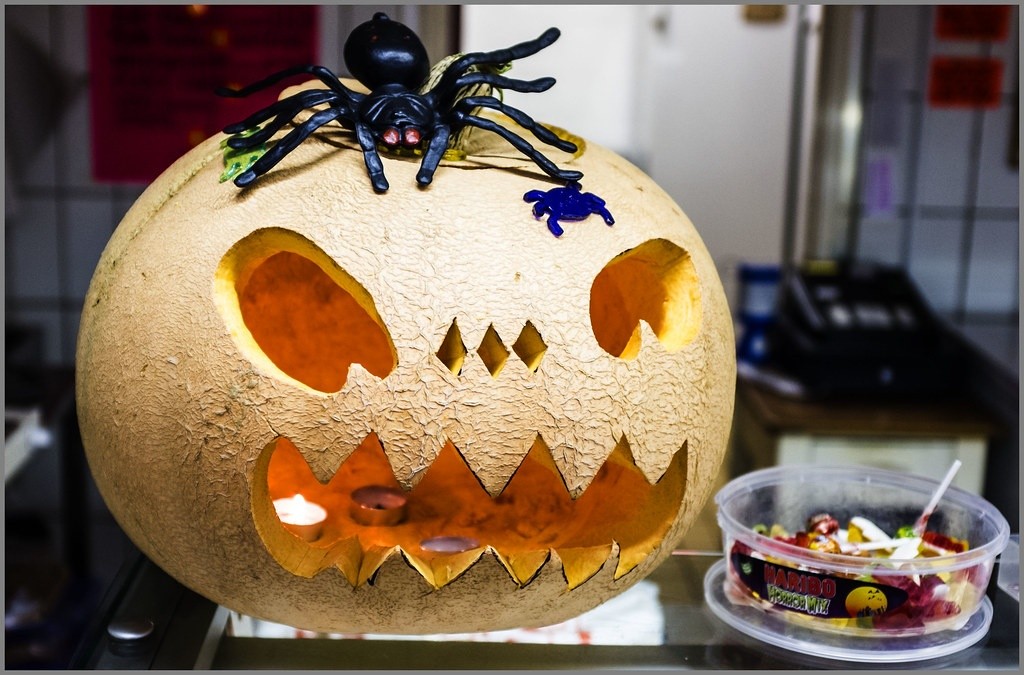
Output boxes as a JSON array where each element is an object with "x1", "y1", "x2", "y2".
[{"x1": 712, "y1": 463, "x2": 1011, "y2": 638}]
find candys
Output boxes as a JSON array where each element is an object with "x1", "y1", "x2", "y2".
[{"x1": 724, "y1": 457, "x2": 987, "y2": 629}]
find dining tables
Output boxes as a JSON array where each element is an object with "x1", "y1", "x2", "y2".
[{"x1": 89, "y1": 544, "x2": 1020, "y2": 672}]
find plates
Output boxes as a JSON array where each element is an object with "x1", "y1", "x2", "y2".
[{"x1": 703, "y1": 554, "x2": 995, "y2": 663}]
